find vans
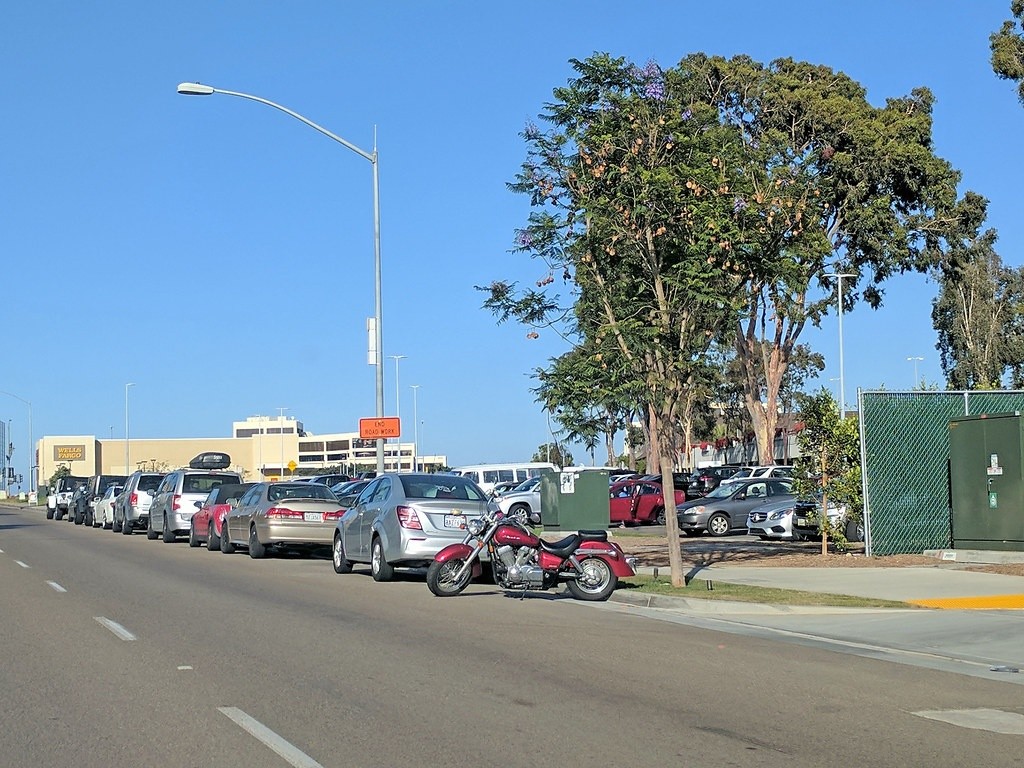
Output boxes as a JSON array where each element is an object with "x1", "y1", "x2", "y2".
[{"x1": 446, "y1": 462, "x2": 558, "y2": 499}]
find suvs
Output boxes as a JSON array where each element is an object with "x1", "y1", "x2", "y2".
[
  {"x1": 111, "y1": 469, "x2": 172, "y2": 536},
  {"x1": 46, "y1": 474, "x2": 129, "y2": 527},
  {"x1": 146, "y1": 452, "x2": 244, "y2": 543}
]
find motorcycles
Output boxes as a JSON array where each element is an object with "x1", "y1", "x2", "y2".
[{"x1": 426, "y1": 476, "x2": 638, "y2": 602}]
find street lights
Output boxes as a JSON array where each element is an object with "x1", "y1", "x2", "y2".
[
  {"x1": 276, "y1": 408, "x2": 290, "y2": 480},
  {"x1": 907, "y1": 356, "x2": 924, "y2": 388},
  {"x1": 257, "y1": 415, "x2": 264, "y2": 482},
  {"x1": 175, "y1": 82, "x2": 384, "y2": 477},
  {"x1": 125, "y1": 383, "x2": 137, "y2": 477},
  {"x1": 829, "y1": 378, "x2": 841, "y2": 404},
  {"x1": 409, "y1": 384, "x2": 422, "y2": 473},
  {"x1": 821, "y1": 271, "x2": 858, "y2": 420},
  {"x1": 387, "y1": 355, "x2": 408, "y2": 474}
]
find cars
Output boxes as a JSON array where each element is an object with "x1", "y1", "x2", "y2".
[
  {"x1": 484, "y1": 465, "x2": 865, "y2": 543},
  {"x1": 189, "y1": 482, "x2": 278, "y2": 551},
  {"x1": 609, "y1": 479, "x2": 685, "y2": 526},
  {"x1": 676, "y1": 476, "x2": 799, "y2": 537},
  {"x1": 277, "y1": 471, "x2": 377, "y2": 504},
  {"x1": 92, "y1": 486, "x2": 124, "y2": 530},
  {"x1": 332, "y1": 474, "x2": 502, "y2": 582},
  {"x1": 220, "y1": 480, "x2": 349, "y2": 559}
]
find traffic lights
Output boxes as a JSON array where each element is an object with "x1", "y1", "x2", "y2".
[
  {"x1": 14, "y1": 475, "x2": 16, "y2": 482},
  {"x1": 2, "y1": 467, "x2": 6, "y2": 478},
  {"x1": 19, "y1": 474, "x2": 23, "y2": 483}
]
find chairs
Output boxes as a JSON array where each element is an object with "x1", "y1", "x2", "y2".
[
  {"x1": 295, "y1": 489, "x2": 309, "y2": 498},
  {"x1": 409, "y1": 486, "x2": 423, "y2": 497},
  {"x1": 212, "y1": 482, "x2": 221, "y2": 489},
  {"x1": 436, "y1": 490, "x2": 457, "y2": 497}
]
